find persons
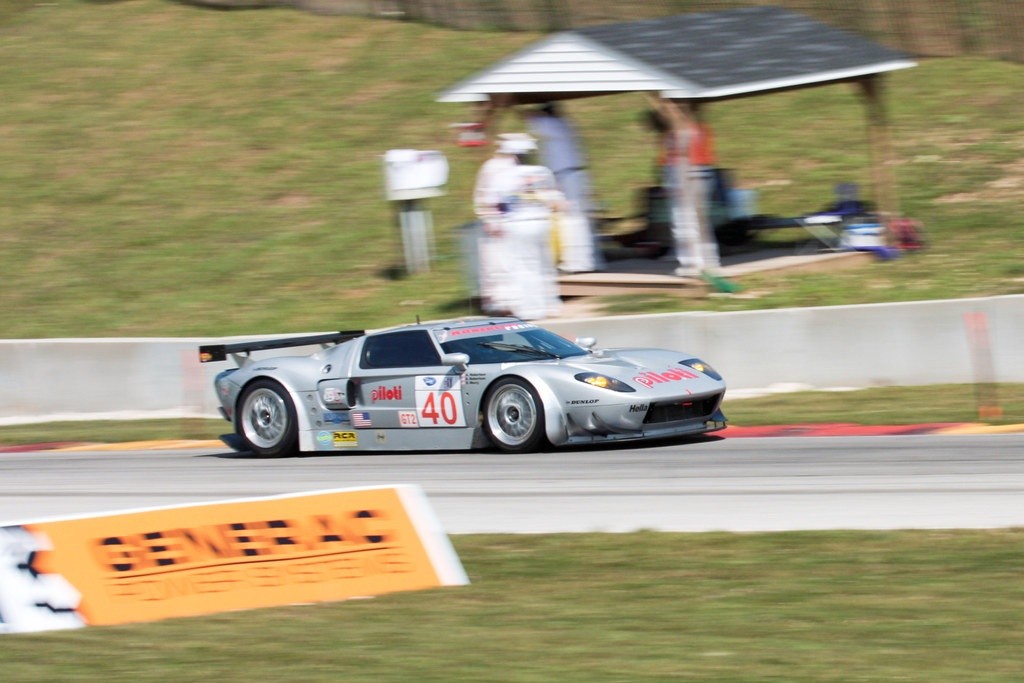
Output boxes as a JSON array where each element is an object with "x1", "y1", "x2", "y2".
[
  {"x1": 640, "y1": 95, "x2": 723, "y2": 280},
  {"x1": 473, "y1": 100, "x2": 610, "y2": 320}
]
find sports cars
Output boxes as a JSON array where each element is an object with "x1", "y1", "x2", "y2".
[{"x1": 196, "y1": 316, "x2": 729, "y2": 456}]
